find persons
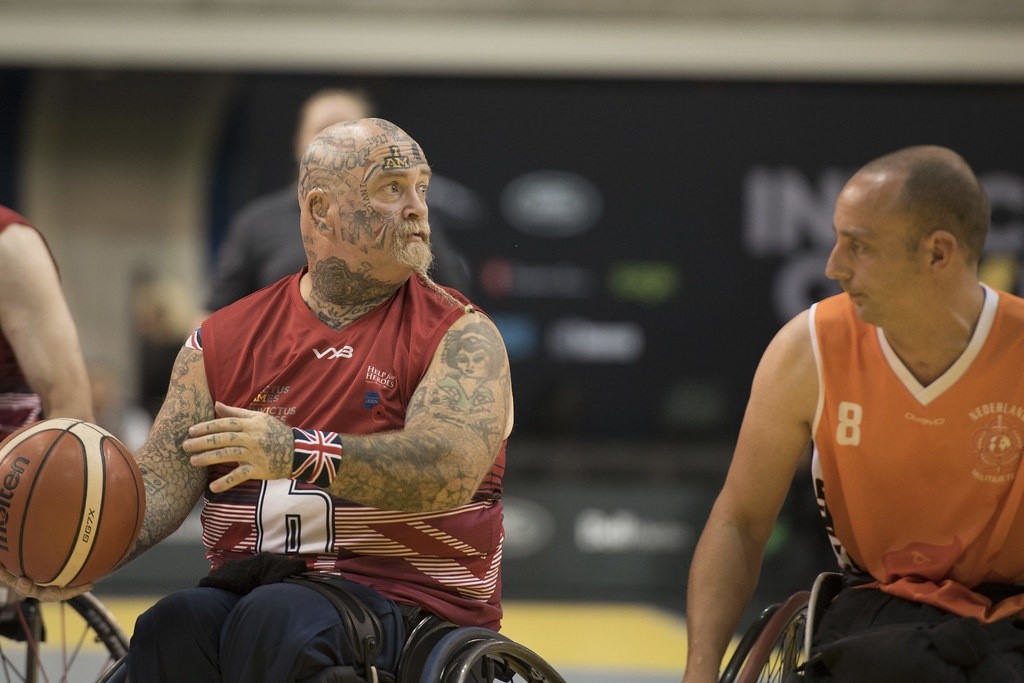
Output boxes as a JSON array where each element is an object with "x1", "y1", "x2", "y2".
[
  {"x1": 0, "y1": 88, "x2": 517, "y2": 683},
  {"x1": 683, "y1": 146, "x2": 1024, "y2": 682}
]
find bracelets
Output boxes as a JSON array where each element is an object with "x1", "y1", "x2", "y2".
[{"x1": 291, "y1": 426, "x2": 343, "y2": 488}]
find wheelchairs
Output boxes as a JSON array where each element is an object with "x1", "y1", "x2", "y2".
[
  {"x1": 0, "y1": 583, "x2": 569, "y2": 683},
  {"x1": 719, "y1": 566, "x2": 843, "y2": 683}
]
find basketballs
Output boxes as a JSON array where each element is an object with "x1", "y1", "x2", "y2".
[{"x1": 0, "y1": 416, "x2": 146, "y2": 588}]
